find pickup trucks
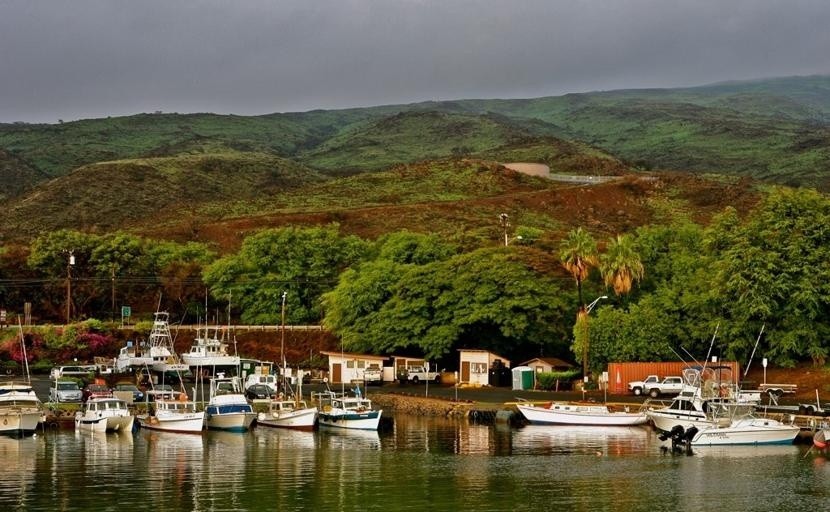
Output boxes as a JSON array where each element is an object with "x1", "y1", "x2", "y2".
[
  {"x1": 629, "y1": 375, "x2": 696, "y2": 398},
  {"x1": 398, "y1": 365, "x2": 441, "y2": 384}
]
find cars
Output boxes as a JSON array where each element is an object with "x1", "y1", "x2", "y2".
[
  {"x1": 209, "y1": 375, "x2": 277, "y2": 399},
  {"x1": 363, "y1": 367, "x2": 384, "y2": 386},
  {"x1": 48, "y1": 381, "x2": 176, "y2": 403}
]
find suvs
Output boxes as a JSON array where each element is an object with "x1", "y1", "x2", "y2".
[{"x1": 49, "y1": 365, "x2": 92, "y2": 383}]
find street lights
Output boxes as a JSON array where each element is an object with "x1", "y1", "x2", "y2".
[
  {"x1": 583, "y1": 295, "x2": 609, "y2": 383},
  {"x1": 281, "y1": 292, "x2": 287, "y2": 369},
  {"x1": 66, "y1": 252, "x2": 76, "y2": 326},
  {"x1": 762, "y1": 358, "x2": 768, "y2": 384},
  {"x1": 504, "y1": 234, "x2": 523, "y2": 246},
  {"x1": 712, "y1": 355, "x2": 718, "y2": 372}
]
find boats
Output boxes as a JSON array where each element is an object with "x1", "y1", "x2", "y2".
[
  {"x1": 0, "y1": 384, "x2": 45, "y2": 435},
  {"x1": 136, "y1": 391, "x2": 206, "y2": 432},
  {"x1": 319, "y1": 391, "x2": 383, "y2": 431},
  {"x1": 516, "y1": 402, "x2": 649, "y2": 426},
  {"x1": 114, "y1": 310, "x2": 240, "y2": 376},
  {"x1": 74, "y1": 393, "x2": 135, "y2": 435},
  {"x1": 203, "y1": 377, "x2": 258, "y2": 433},
  {"x1": 257, "y1": 401, "x2": 319, "y2": 432},
  {"x1": 648, "y1": 359, "x2": 802, "y2": 446}
]
